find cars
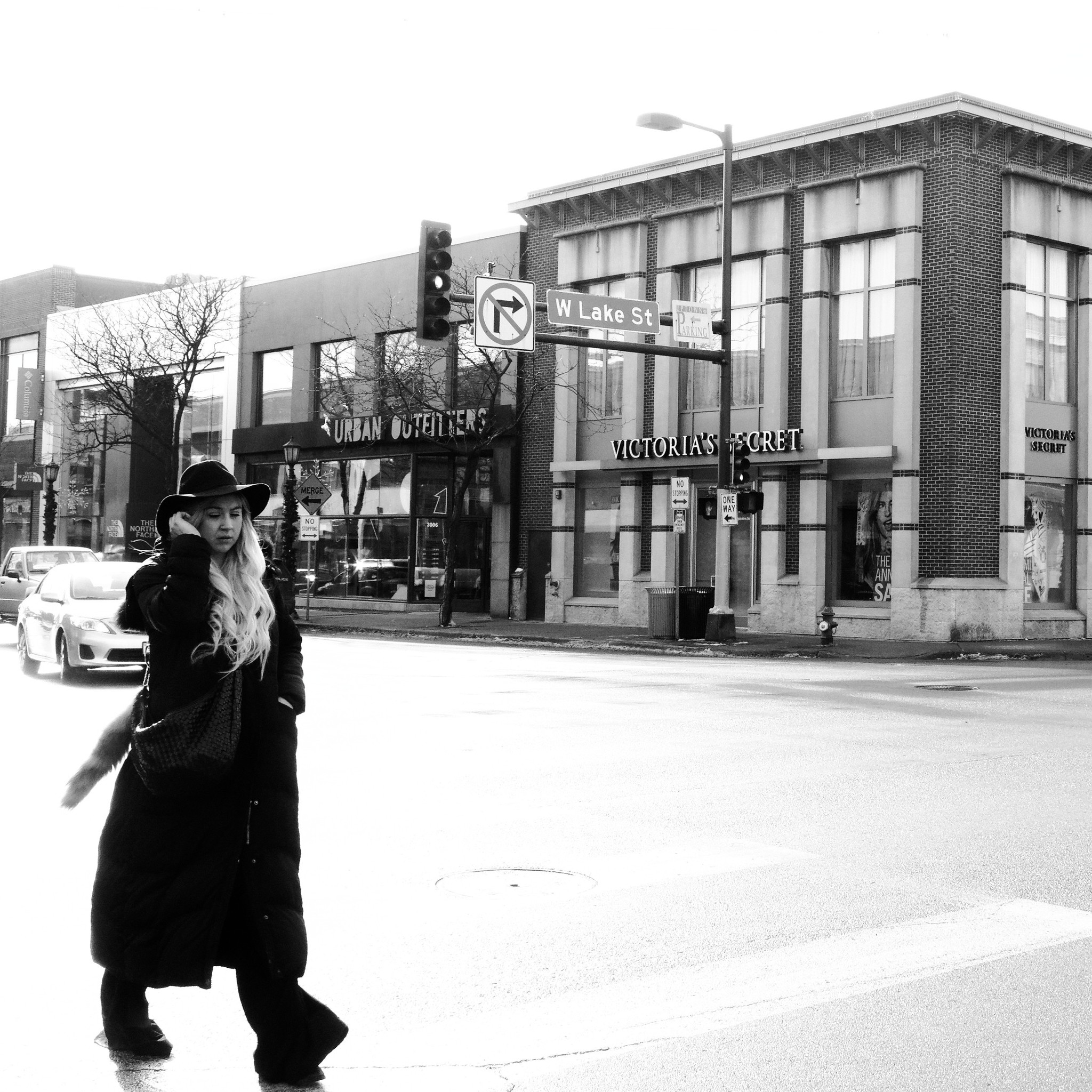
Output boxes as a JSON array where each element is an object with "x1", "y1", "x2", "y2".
[{"x1": 16, "y1": 561, "x2": 161, "y2": 682}]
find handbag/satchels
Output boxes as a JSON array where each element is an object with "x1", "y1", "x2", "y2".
[{"x1": 133, "y1": 661, "x2": 242, "y2": 793}]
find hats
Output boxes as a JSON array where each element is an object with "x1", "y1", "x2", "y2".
[{"x1": 154, "y1": 459, "x2": 271, "y2": 538}]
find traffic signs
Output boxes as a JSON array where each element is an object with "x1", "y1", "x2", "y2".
[
  {"x1": 670, "y1": 474, "x2": 690, "y2": 510},
  {"x1": 292, "y1": 474, "x2": 333, "y2": 515}
]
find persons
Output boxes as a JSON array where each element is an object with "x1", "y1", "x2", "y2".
[
  {"x1": 855, "y1": 492, "x2": 892, "y2": 600},
  {"x1": 65, "y1": 462, "x2": 348, "y2": 1086}
]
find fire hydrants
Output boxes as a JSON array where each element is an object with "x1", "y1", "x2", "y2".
[{"x1": 817, "y1": 606, "x2": 838, "y2": 645}]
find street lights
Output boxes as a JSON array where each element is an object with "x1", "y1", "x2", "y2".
[
  {"x1": 636, "y1": 109, "x2": 734, "y2": 639},
  {"x1": 277, "y1": 435, "x2": 300, "y2": 617},
  {"x1": 43, "y1": 457, "x2": 59, "y2": 544}
]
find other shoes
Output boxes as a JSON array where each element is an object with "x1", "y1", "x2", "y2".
[
  {"x1": 254, "y1": 1061, "x2": 325, "y2": 1086},
  {"x1": 106, "y1": 1035, "x2": 174, "y2": 1059}
]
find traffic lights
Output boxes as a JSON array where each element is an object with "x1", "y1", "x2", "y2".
[
  {"x1": 741, "y1": 490, "x2": 764, "y2": 514},
  {"x1": 732, "y1": 441, "x2": 750, "y2": 486},
  {"x1": 696, "y1": 497, "x2": 717, "y2": 520}
]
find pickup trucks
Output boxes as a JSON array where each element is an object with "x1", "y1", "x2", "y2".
[{"x1": 0, "y1": 545, "x2": 103, "y2": 621}]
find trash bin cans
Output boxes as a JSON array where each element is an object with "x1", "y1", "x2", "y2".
[
  {"x1": 644, "y1": 586, "x2": 676, "y2": 639},
  {"x1": 679, "y1": 585, "x2": 715, "y2": 639}
]
